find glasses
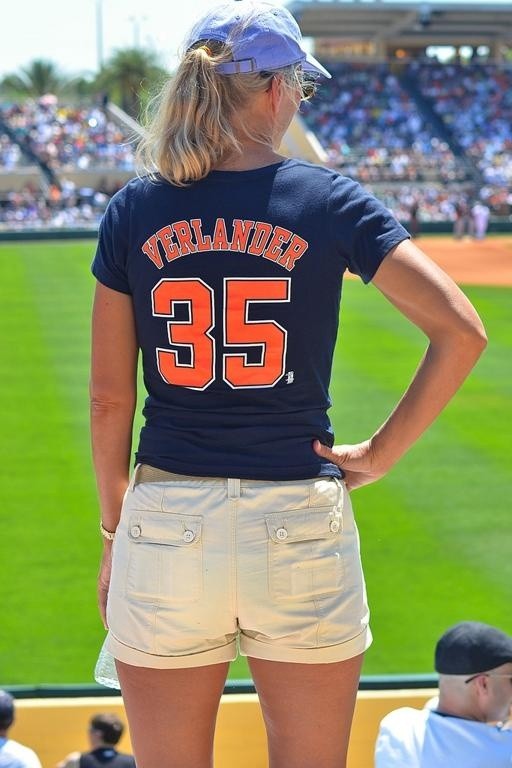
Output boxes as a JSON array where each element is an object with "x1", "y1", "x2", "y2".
[{"x1": 260, "y1": 69, "x2": 316, "y2": 101}]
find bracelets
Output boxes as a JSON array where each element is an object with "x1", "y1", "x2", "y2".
[{"x1": 99, "y1": 521, "x2": 119, "y2": 541}]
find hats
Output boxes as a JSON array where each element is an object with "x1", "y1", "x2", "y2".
[
  {"x1": 434, "y1": 623, "x2": 511, "y2": 675},
  {"x1": 186, "y1": 0, "x2": 332, "y2": 80}
]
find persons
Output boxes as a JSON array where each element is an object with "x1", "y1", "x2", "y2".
[
  {"x1": 0, "y1": 692, "x2": 44, "y2": 768},
  {"x1": 375, "y1": 621, "x2": 512, "y2": 768},
  {"x1": 1, "y1": 90, "x2": 147, "y2": 233},
  {"x1": 54, "y1": 714, "x2": 136, "y2": 768},
  {"x1": 299, "y1": 48, "x2": 510, "y2": 241},
  {"x1": 88, "y1": 0, "x2": 489, "y2": 767}
]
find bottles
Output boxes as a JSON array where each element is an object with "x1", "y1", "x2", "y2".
[{"x1": 92, "y1": 624, "x2": 123, "y2": 691}]
